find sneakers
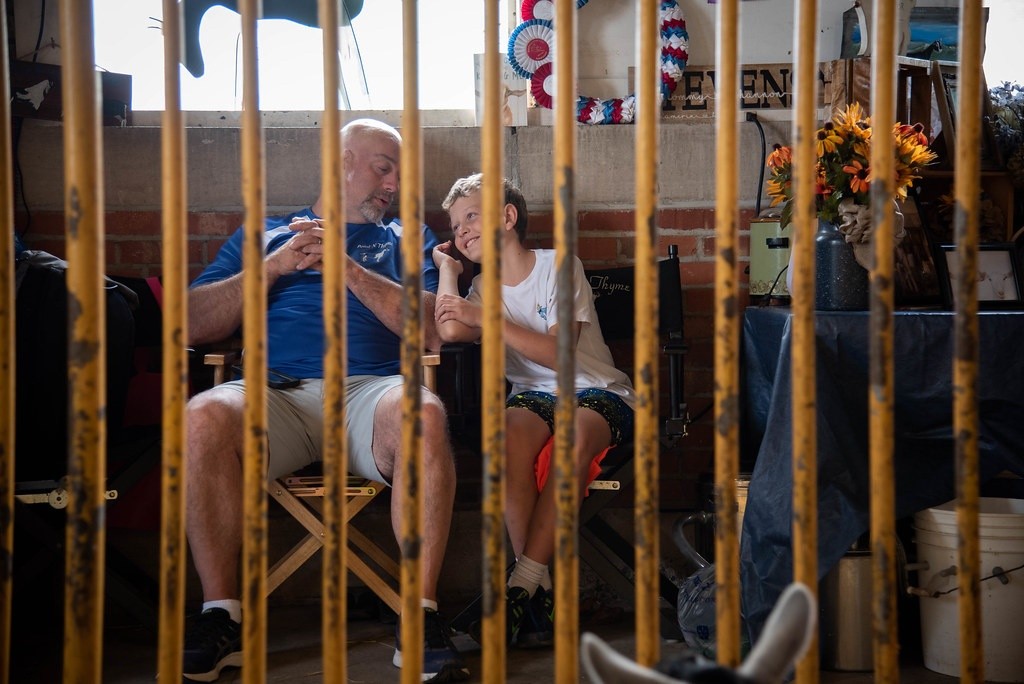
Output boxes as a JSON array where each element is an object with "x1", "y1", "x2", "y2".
[
  {"x1": 524, "y1": 584, "x2": 554, "y2": 644},
  {"x1": 184, "y1": 608, "x2": 244, "y2": 681},
  {"x1": 452, "y1": 586, "x2": 528, "y2": 645},
  {"x1": 393, "y1": 606, "x2": 470, "y2": 681}
]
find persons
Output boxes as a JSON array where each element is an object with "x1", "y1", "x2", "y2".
[
  {"x1": 182, "y1": 118, "x2": 469, "y2": 680},
  {"x1": 432, "y1": 173, "x2": 636, "y2": 645}
]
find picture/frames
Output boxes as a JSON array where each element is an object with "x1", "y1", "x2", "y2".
[
  {"x1": 940, "y1": 246, "x2": 1024, "y2": 311},
  {"x1": 933, "y1": 60, "x2": 1004, "y2": 171}
]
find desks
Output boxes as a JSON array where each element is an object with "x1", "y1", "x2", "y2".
[{"x1": 740, "y1": 302, "x2": 1024, "y2": 631}]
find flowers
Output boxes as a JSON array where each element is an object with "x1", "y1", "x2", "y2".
[
  {"x1": 984, "y1": 80, "x2": 1024, "y2": 150},
  {"x1": 765, "y1": 101, "x2": 940, "y2": 225}
]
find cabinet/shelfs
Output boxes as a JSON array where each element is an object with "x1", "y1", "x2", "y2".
[{"x1": 832, "y1": 55, "x2": 1014, "y2": 241}]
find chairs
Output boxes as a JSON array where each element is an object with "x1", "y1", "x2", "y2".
[
  {"x1": 440, "y1": 244, "x2": 710, "y2": 639},
  {"x1": 204, "y1": 326, "x2": 458, "y2": 621},
  {"x1": 15, "y1": 249, "x2": 161, "y2": 607}
]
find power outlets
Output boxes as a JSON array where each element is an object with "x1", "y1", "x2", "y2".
[{"x1": 745, "y1": 111, "x2": 758, "y2": 121}]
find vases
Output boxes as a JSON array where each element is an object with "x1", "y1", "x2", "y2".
[{"x1": 815, "y1": 217, "x2": 869, "y2": 310}]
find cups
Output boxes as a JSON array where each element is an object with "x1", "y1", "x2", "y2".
[{"x1": 942, "y1": 242, "x2": 1020, "y2": 311}]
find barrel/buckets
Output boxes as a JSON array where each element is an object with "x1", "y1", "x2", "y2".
[
  {"x1": 905, "y1": 497, "x2": 1024, "y2": 682},
  {"x1": 821, "y1": 550, "x2": 874, "y2": 671}
]
